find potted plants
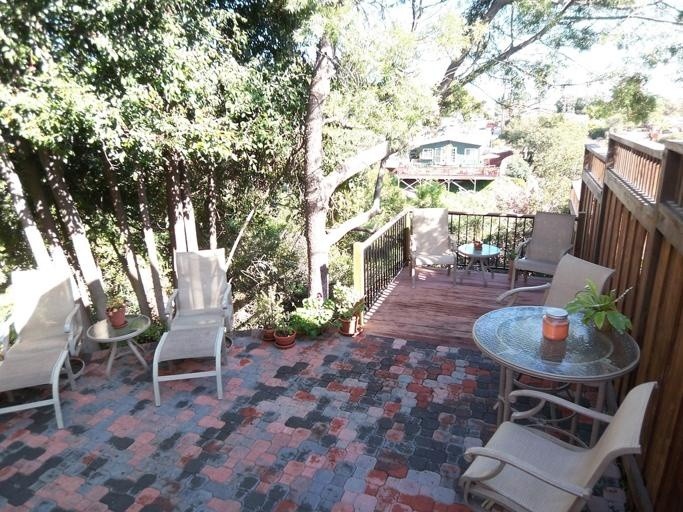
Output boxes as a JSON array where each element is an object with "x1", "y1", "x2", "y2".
[
  {"x1": 569, "y1": 282, "x2": 632, "y2": 335},
  {"x1": 260, "y1": 302, "x2": 276, "y2": 342},
  {"x1": 273, "y1": 311, "x2": 297, "y2": 349},
  {"x1": 106, "y1": 275, "x2": 127, "y2": 328},
  {"x1": 329, "y1": 291, "x2": 365, "y2": 336}
]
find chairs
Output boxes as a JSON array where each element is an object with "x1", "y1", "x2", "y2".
[
  {"x1": 0, "y1": 268, "x2": 81, "y2": 430},
  {"x1": 410, "y1": 209, "x2": 456, "y2": 286},
  {"x1": 460, "y1": 382, "x2": 657, "y2": 511},
  {"x1": 510, "y1": 212, "x2": 574, "y2": 288},
  {"x1": 495, "y1": 254, "x2": 615, "y2": 313},
  {"x1": 152, "y1": 248, "x2": 232, "y2": 406}
]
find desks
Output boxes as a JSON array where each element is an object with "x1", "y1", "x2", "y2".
[
  {"x1": 472, "y1": 305, "x2": 640, "y2": 445},
  {"x1": 456, "y1": 243, "x2": 501, "y2": 285},
  {"x1": 86, "y1": 314, "x2": 151, "y2": 376}
]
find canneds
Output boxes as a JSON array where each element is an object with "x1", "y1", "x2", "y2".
[{"x1": 542, "y1": 308, "x2": 570, "y2": 342}]
